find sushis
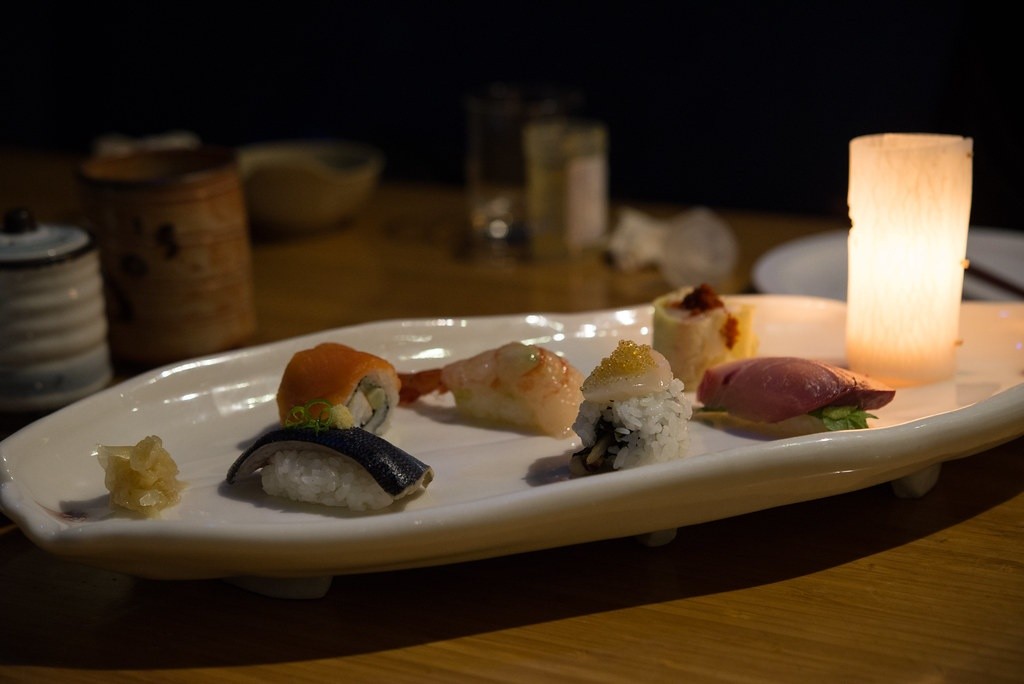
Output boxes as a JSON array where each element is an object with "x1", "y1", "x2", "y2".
[
  {"x1": 277, "y1": 342, "x2": 400, "y2": 435},
  {"x1": 653, "y1": 281, "x2": 758, "y2": 392},
  {"x1": 223, "y1": 425, "x2": 435, "y2": 512},
  {"x1": 573, "y1": 339, "x2": 693, "y2": 471}
]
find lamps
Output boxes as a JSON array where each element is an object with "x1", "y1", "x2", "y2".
[{"x1": 841, "y1": 132, "x2": 974, "y2": 392}]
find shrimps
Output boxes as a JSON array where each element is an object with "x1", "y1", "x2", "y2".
[{"x1": 397, "y1": 342, "x2": 586, "y2": 437}]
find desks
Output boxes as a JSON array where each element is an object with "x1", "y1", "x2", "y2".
[{"x1": 0, "y1": 179, "x2": 1024, "y2": 684}]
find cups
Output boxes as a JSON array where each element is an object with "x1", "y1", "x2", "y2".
[
  {"x1": 520, "y1": 119, "x2": 611, "y2": 259},
  {"x1": 84, "y1": 144, "x2": 257, "y2": 361},
  {"x1": 464, "y1": 95, "x2": 541, "y2": 249},
  {"x1": 840, "y1": 131, "x2": 976, "y2": 384}
]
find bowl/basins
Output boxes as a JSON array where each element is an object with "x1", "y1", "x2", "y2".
[{"x1": 241, "y1": 146, "x2": 384, "y2": 238}]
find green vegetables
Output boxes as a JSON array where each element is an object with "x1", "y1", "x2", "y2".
[{"x1": 807, "y1": 396, "x2": 879, "y2": 431}]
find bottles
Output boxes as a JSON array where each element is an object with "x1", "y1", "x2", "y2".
[{"x1": 1, "y1": 188, "x2": 113, "y2": 412}]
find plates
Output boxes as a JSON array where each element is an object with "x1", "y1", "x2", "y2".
[
  {"x1": 0, "y1": 292, "x2": 1024, "y2": 573},
  {"x1": 752, "y1": 227, "x2": 1024, "y2": 300}
]
